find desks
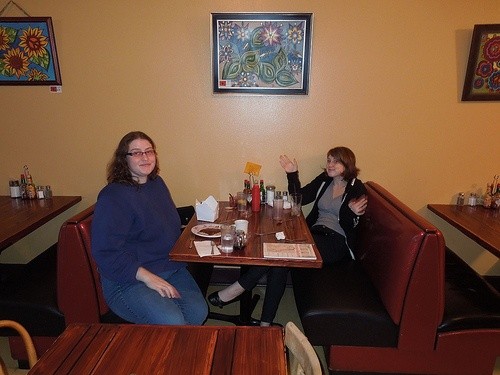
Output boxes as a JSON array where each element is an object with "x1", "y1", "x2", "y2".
[
  {"x1": 427, "y1": 203, "x2": 500, "y2": 261},
  {"x1": 26, "y1": 323, "x2": 287, "y2": 375},
  {"x1": 0, "y1": 196, "x2": 82, "y2": 253}
]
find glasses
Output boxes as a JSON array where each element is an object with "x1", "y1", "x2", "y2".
[{"x1": 126, "y1": 149, "x2": 154, "y2": 157}]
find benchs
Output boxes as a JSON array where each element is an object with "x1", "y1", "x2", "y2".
[{"x1": 0, "y1": 180, "x2": 500, "y2": 375}]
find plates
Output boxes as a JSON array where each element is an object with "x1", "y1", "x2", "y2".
[{"x1": 191, "y1": 223, "x2": 226, "y2": 238}]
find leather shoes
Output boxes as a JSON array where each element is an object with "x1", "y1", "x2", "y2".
[{"x1": 208, "y1": 290, "x2": 244, "y2": 305}]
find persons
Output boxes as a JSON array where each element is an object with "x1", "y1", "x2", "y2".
[
  {"x1": 91, "y1": 131, "x2": 208, "y2": 325},
  {"x1": 208, "y1": 146, "x2": 369, "y2": 327}
]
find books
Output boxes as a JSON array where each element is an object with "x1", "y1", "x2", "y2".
[{"x1": 264, "y1": 244, "x2": 317, "y2": 260}]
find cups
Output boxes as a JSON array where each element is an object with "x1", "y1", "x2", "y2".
[
  {"x1": 272, "y1": 199, "x2": 285, "y2": 221},
  {"x1": 290, "y1": 193, "x2": 302, "y2": 217},
  {"x1": 220, "y1": 225, "x2": 236, "y2": 253},
  {"x1": 234, "y1": 220, "x2": 249, "y2": 232},
  {"x1": 237, "y1": 192, "x2": 247, "y2": 211}
]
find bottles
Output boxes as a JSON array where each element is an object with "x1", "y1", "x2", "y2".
[
  {"x1": 243, "y1": 179, "x2": 288, "y2": 212},
  {"x1": 468, "y1": 192, "x2": 478, "y2": 206},
  {"x1": 20, "y1": 174, "x2": 29, "y2": 200},
  {"x1": 36, "y1": 185, "x2": 46, "y2": 199},
  {"x1": 456, "y1": 192, "x2": 466, "y2": 206},
  {"x1": 44, "y1": 185, "x2": 53, "y2": 200},
  {"x1": 26, "y1": 174, "x2": 38, "y2": 200},
  {"x1": 492, "y1": 184, "x2": 500, "y2": 209},
  {"x1": 9, "y1": 180, "x2": 21, "y2": 199},
  {"x1": 482, "y1": 183, "x2": 492, "y2": 209}
]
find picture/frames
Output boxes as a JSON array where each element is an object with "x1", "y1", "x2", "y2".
[
  {"x1": 210, "y1": 11, "x2": 314, "y2": 96},
  {"x1": 461, "y1": 23, "x2": 500, "y2": 101},
  {"x1": 0, "y1": 16, "x2": 63, "y2": 87}
]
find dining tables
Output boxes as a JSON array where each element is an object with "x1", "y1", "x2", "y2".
[{"x1": 167, "y1": 201, "x2": 323, "y2": 326}]
